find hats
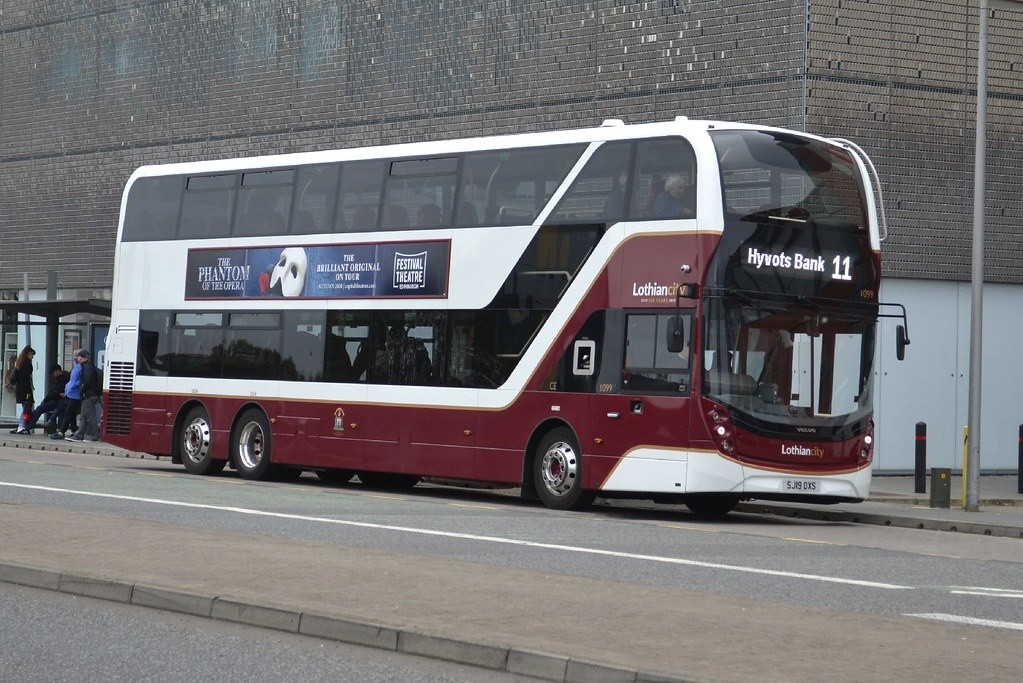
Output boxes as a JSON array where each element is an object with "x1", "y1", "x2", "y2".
[
  {"x1": 49, "y1": 364, "x2": 60, "y2": 374},
  {"x1": 75, "y1": 350, "x2": 90, "y2": 358}
]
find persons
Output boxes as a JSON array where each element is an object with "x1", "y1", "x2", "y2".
[
  {"x1": 8, "y1": 345, "x2": 99, "y2": 442},
  {"x1": 651, "y1": 174, "x2": 694, "y2": 219},
  {"x1": 646, "y1": 339, "x2": 711, "y2": 395}
]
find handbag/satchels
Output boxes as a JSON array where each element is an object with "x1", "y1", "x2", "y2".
[{"x1": 22, "y1": 400, "x2": 34, "y2": 421}]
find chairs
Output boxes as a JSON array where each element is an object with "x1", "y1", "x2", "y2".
[
  {"x1": 416, "y1": 203, "x2": 441, "y2": 227},
  {"x1": 258, "y1": 212, "x2": 285, "y2": 234},
  {"x1": 384, "y1": 204, "x2": 410, "y2": 229},
  {"x1": 642, "y1": 176, "x2": 666, "y2": 219},
  {"x1": 457, "y1": 200, "x2": 478, "y2": 225},
  {"x1": 207, "y1": 214, "x2": 228, "y2": 234},
  {"x1": 331, "y1": 336, "x2": 351, "y2": 378},
  {"x1": 494, "y1": 206, "x2": 537, "y2": 224},
  {"x1": 352, "y1": 207, "x2": 376, "y2": 230},
  {"x1": 293, "y1": 210, "x2": 319, "y2": 233},
  {"x1": 319, "y1": 208, "x2": 348, "y2": 233}
]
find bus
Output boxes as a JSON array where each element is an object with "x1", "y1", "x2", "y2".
[{"x1": 97, "y1": 115, "x2": 911, "y2": 515}]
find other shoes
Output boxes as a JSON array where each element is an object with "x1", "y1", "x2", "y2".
[
  {"x1": 50, "y1": 433, "x2": 64, "y2": 439},
  {"x1": 41, "y1": 421, "x2": 54, "y2": 430},
  {"x1": 11, "y1": 428, "x2": 30, "y2": 434},
  {"x1": 69, "y1": 434, "x2": 75, "y2": 437}
]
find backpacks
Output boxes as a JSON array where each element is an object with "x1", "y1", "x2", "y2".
[{"x1": 82, "y1": 362, "x2": 103, "y2": 397}]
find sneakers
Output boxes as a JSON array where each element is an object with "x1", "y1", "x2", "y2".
[
  {"x1": 65, "y1": 436, "x2": 81, "y2": 442},
  {"x1": 83, "y1": 436, "x2": 99, "y2": 443}
]
